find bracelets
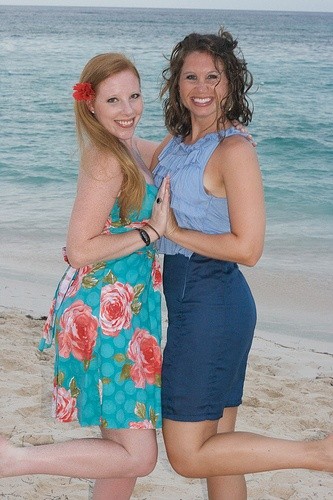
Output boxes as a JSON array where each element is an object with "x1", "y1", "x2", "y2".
[{"x1": 143, "y1": 220, "x2": 162, "y2": 240}]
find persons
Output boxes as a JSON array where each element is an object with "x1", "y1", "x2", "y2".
[
  {"x1": 0, "y1": 53, "x2": 170, "y2": 499},
  {"x1": 149, "y1": 30, "x2": 333, "y2": 499}
]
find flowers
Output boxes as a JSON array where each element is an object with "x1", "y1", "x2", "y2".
[{"x1": 71, "y1": 81, "x2": 96, "y2": 100}]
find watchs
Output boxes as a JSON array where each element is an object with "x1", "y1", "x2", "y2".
[{"x1": 134, "y1": 225, "x2": 151, "y2": 246}]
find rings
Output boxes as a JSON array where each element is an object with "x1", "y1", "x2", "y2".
[{"x1": 156, "y1": 196, "x2": 163, "y2": 204}]
